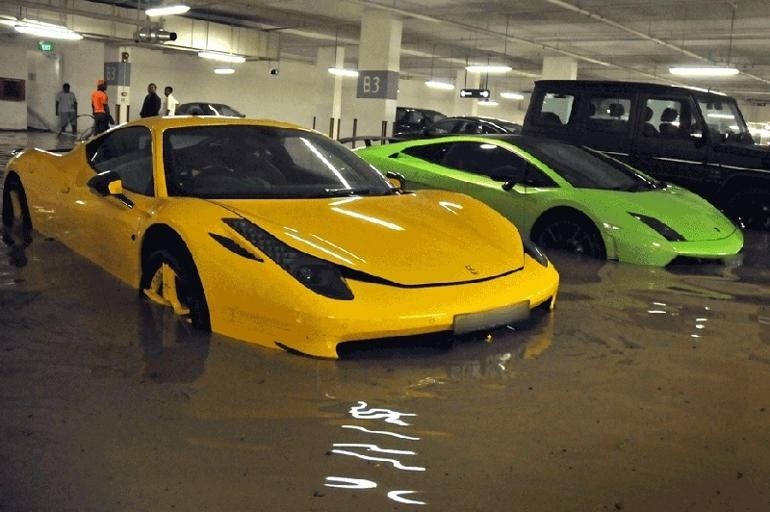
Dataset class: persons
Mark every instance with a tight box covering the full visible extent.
[163,87,179,116]
[140,83,161,118]
[91,79,111,135]
[55,83,78,135]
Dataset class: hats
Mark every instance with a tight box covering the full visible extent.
[97,79,105,86]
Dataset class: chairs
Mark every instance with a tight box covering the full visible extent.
[587,103,680,137]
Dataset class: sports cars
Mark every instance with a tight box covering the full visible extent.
[351,137,745,267]
[1,116,560,360]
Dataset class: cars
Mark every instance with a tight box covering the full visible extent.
[175,102,246,118]
[388,107,523,143]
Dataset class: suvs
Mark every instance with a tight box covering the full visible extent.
[520,80,770,233]
